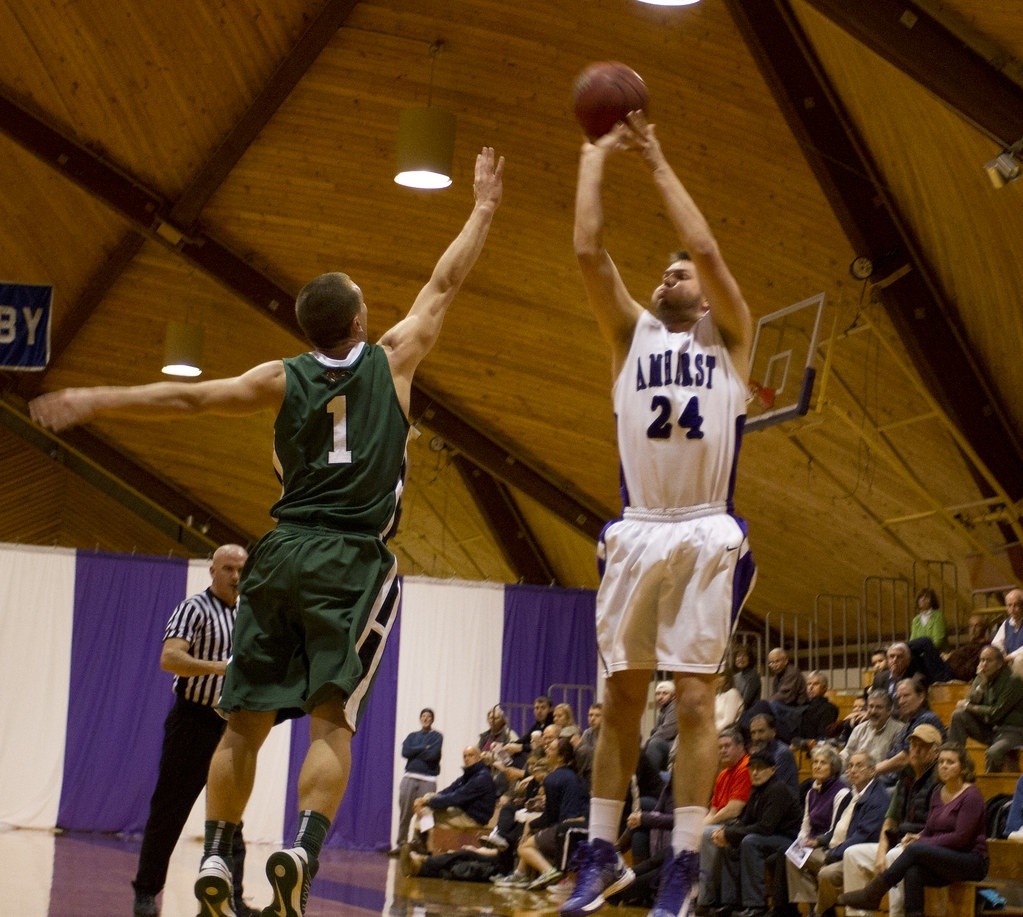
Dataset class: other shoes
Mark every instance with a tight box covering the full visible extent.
[733,907,770,917]
[837,889,880,910]
[704,905,737,917]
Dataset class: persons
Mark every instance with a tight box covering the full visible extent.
[29,148,504,917]
[390,696,662,891]
[133,544,262,917]
[562,108,750,917]
[618,589,1023,917]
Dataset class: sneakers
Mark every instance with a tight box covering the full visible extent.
[530,867,561,890]
[192,851,242,915]
[645,842,702,916]
[257,847,311,913]
[493,871,529,889]
[562,839,638,917]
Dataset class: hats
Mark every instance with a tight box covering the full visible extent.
[905,723,942,746]
[654,681,676,693]
[745,750,775,767]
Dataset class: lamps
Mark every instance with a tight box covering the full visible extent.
[159,300,203,377]
[850,254,873,280]
[409,420,421,439]
[392,38,458,189]
[985,137,1023,189]
[429,437,446,452]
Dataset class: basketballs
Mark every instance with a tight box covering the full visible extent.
[572,60,652,142]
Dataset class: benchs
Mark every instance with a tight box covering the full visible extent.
[795,604,1023,917]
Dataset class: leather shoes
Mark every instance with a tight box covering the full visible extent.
[133,893,156,916]
